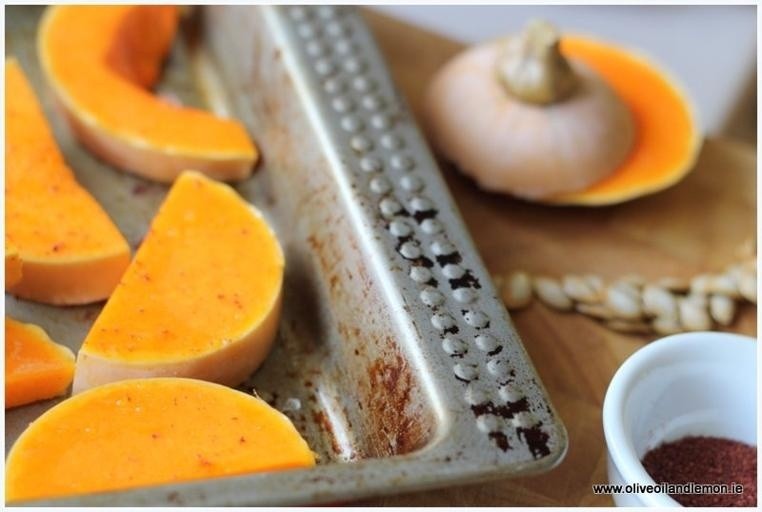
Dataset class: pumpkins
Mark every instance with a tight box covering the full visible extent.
[419,22,703,207]
[5,5,317,500]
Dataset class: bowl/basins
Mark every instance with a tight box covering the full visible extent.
[599,328,758,507]
